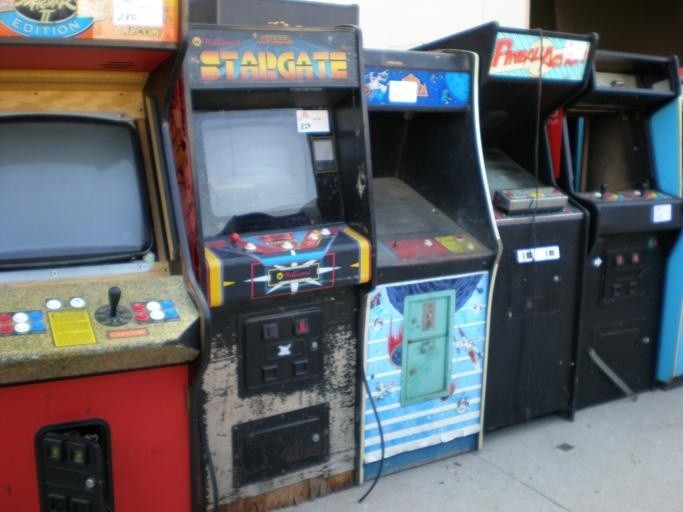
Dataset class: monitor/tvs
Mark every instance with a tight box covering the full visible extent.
[0,112,160,285]
[369,179,455,241]
[581,114,648,192]
[197,113,317,220]
[482,150,534,193]
